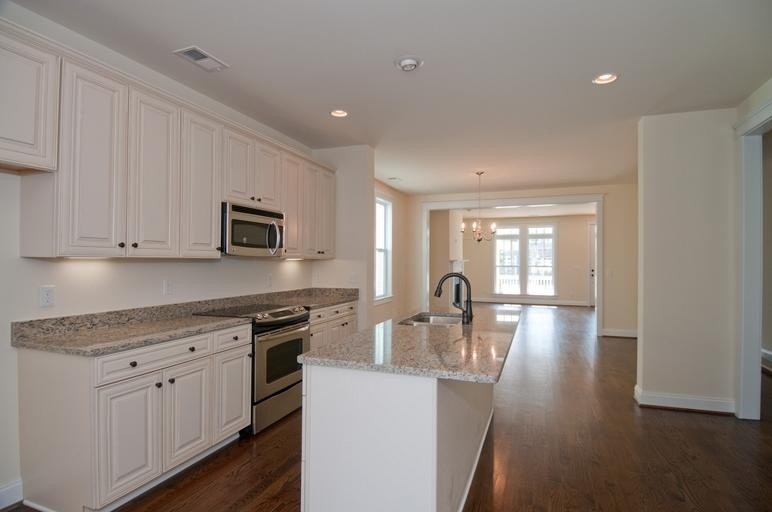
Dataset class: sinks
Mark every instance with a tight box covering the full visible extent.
[398,311,473,326]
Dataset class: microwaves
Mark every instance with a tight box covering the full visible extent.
[220,201,287,259]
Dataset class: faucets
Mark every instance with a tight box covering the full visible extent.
[433,325,473,372]
[434,272,473,322]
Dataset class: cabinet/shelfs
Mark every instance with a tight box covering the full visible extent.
[222,126,283,212]
[280,147,299,258]
[299,157,337,261]
[21,54,179,261]
[16,332,209,510]
[180,108,221,262]
[211,322,253,452]
[309,299,360,348]
[2,22,63,175]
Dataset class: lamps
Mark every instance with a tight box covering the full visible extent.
[458,172,497,247]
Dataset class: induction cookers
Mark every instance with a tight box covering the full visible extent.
[193,302,311,334]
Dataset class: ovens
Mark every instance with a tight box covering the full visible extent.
[252,321,310,434]
